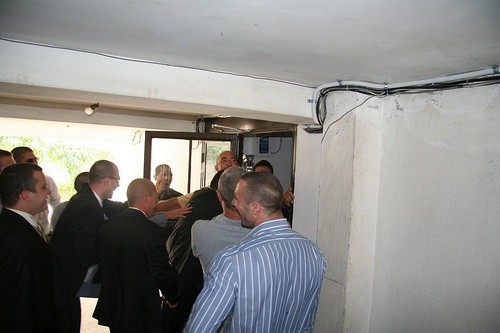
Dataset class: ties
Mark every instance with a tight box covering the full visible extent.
[37,221,48,244]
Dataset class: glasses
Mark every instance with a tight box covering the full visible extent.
[102,175,120,182]
[26,156,38,163]
[160,173,173,177]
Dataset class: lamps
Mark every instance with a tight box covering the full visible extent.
[84,103,100,116]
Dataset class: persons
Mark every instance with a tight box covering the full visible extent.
[92,178,193,333]
[192,167,252,292]
[283,187,294,207]
[182,172,326,333]
[11,146,60,240]
[0,162,81,300]
[45,171,98,241]
[215,150,240,171]
[160,170,224,333]
[49,160,130,285]
[252,160,275,177]
[152,164,184,200]
[0,149,16,171]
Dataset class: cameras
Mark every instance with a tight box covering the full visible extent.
[243,154,254,172]
